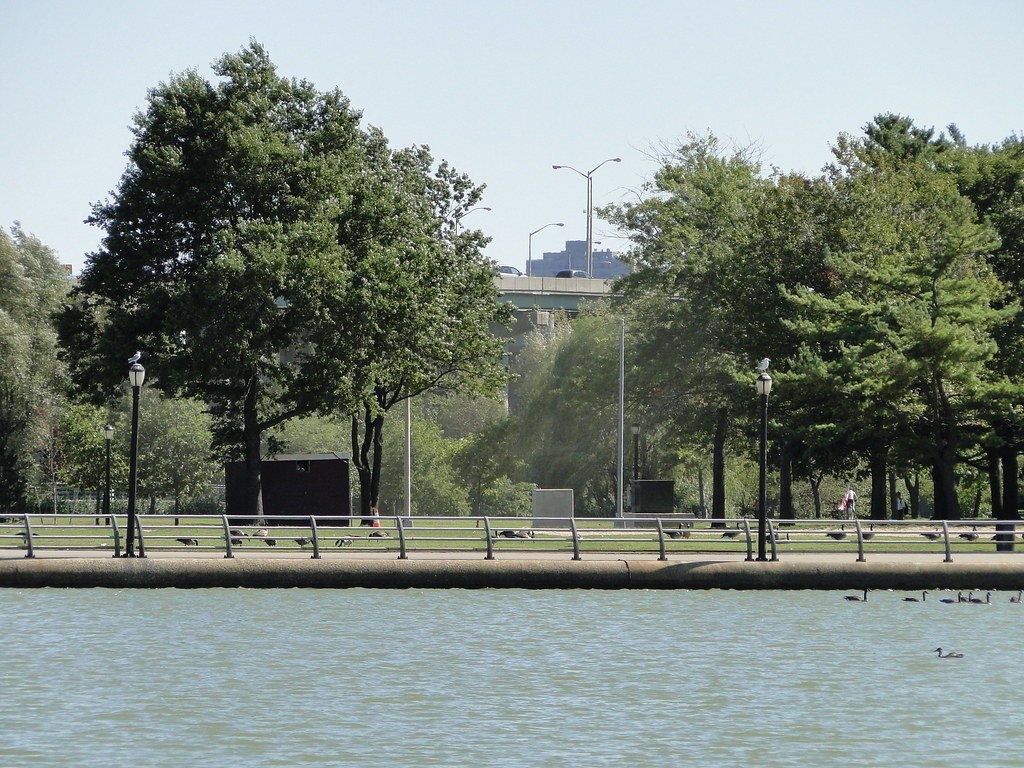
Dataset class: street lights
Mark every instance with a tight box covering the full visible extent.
[102,423,116,525]
[552,156,623,278]
[122,351,147,560]
[453,205,492,236]
[527,221,564,276]
[753,357,774,563]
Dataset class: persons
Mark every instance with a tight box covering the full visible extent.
[844,487,858,520]
[896,491,906,520]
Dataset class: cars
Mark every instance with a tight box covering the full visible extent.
[556,268,592,277]
[493,265,525,278]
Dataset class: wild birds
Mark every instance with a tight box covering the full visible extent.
[843,587,872,602]
[939,591,963,604]
[663,522,691,539]
[826,523,846,540]
[252,522,269,537]
[766,526,791,540]
[492,530,535,547]
[991,535,996,541]
[1010,590,1024,603]
[958,526,979,542]
[224,529,251,547]
[265,539,276,547]
[174,539,198,547]
[292,539,313,547]
[334,534,361,547]
[919,528,941,541]
[960,591,973,603]
[901,591,930,602]
[368,532,387,542]
[721,523,742,540]
[933,648,966,658]
[863,524,875,540]
[971,592,992,604]
